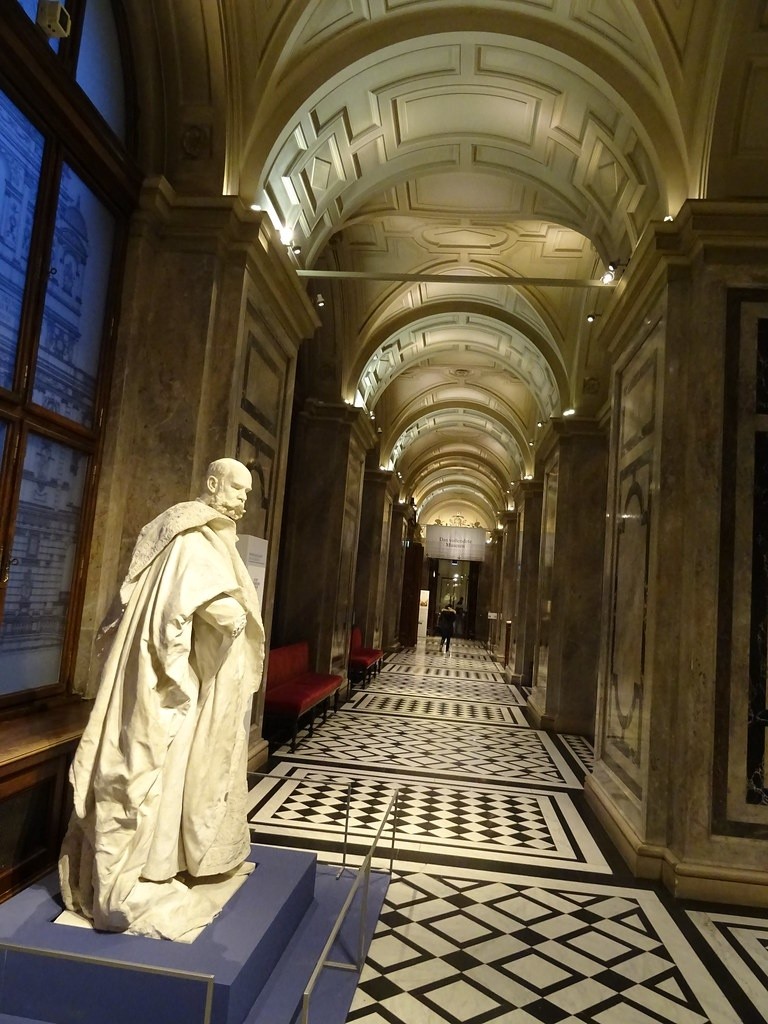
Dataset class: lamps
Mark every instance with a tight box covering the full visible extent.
[608,257,628,272]
[538,419,548,427]
[311,294,325,307]
[285,238,301,254]
[587,310,602,321]
[529,439,539,445]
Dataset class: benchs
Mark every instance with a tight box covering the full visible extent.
[262,642,343,754]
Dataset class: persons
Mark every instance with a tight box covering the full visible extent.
[57,457,265,933]
[438,604,456,658]
[454,597,465,635]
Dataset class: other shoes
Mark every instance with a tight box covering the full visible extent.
[438,645,443,652]
[444,651,450,658]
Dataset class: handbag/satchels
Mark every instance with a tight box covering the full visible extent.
[436,612,442,628]
